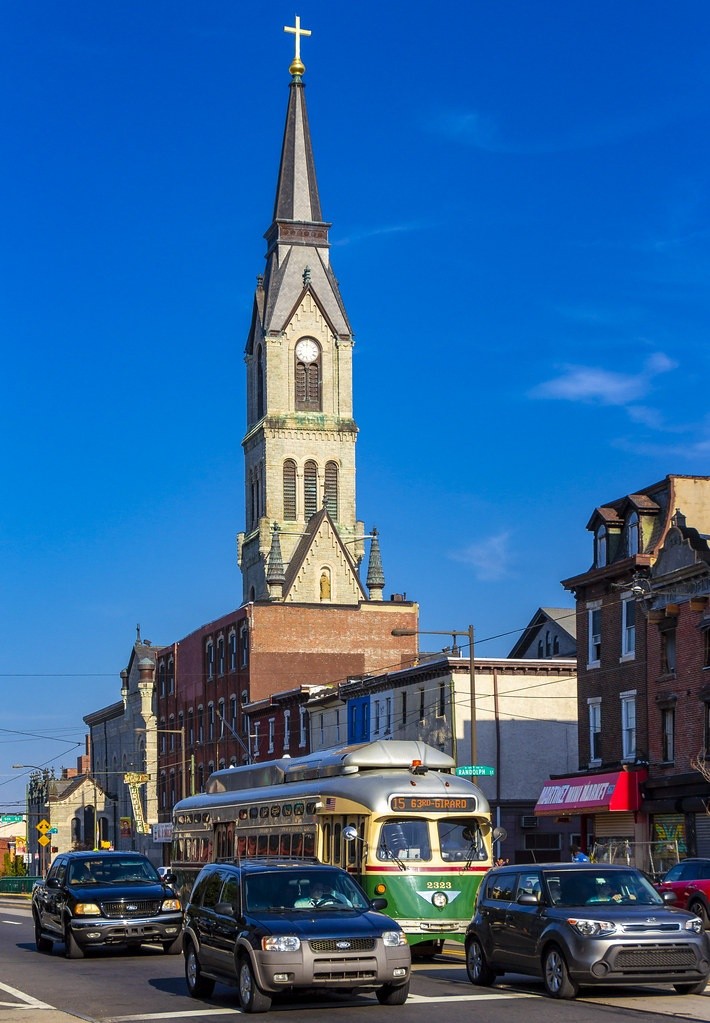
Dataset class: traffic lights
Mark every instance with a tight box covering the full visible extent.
[52,847,58,852]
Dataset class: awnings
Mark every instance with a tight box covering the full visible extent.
[533,771,642,816]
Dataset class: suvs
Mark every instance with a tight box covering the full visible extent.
[31,849,183,959]
[463,861,710,1000]
[180,854,415,1013]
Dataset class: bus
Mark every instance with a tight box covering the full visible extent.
[169,738,508,963]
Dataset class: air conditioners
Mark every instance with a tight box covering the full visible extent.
[520,815,537,827]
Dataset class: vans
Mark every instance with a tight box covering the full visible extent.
[636,857,710,930]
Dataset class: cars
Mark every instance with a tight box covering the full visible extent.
[158,866,172,890]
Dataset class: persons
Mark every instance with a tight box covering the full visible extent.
[294,877,354,908]
[570,845,589,862]
[495,857,510,866]
[71,866,96,883]
[438,826,464,851]
[585,883,636,903]
[120,821,130,834]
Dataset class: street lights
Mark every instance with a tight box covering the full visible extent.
[135,726,185,801]
[390,624,481,784]
[12,764,51,873]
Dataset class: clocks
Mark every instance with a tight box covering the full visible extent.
[295,340,319,363]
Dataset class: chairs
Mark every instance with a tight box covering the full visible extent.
[248,879,273,910]
